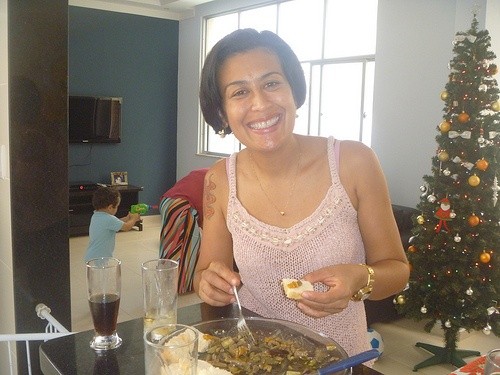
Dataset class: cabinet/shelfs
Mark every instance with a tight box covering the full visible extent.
[69,183,145,237]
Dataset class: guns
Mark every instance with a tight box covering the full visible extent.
[130,204,162,213]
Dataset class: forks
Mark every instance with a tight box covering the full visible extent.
[231,285,258,347]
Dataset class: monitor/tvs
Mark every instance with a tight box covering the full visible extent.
[69,94,122,143]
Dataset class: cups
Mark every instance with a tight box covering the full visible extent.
[141,259,179,340]
[86,256,123,350]
[144,324,198,375]
[484,348,500,375]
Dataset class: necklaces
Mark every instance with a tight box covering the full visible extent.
[245,135,302,216]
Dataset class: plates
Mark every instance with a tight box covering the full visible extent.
[152,316,351,375]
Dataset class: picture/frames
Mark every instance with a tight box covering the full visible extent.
[111,171,128,185]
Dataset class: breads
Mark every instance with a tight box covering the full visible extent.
[282,278,314,300]
[159,325,234,375]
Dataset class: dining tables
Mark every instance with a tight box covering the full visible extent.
[39,296,384,375]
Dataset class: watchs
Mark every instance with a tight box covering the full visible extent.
[351,262,375,302]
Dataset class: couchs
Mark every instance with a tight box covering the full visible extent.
[364,203,424,325]
[159,166,208,297]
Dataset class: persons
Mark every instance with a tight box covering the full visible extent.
[192,27,410,368]
[84,187,140,266]
